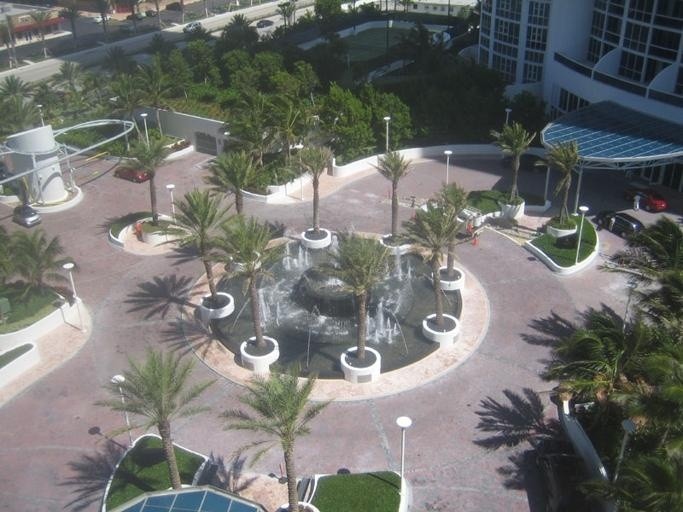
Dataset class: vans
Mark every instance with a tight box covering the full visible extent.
[182,21,202,32]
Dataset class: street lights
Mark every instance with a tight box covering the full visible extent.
[108,373,136,448]
[392,414,414,495]
[136,110,152,152]
[610,416,639,483]
[574,204,590,265]
[383,12,395,65]
[36,102,47,126]
[441,147,454,185]
[59,258,90,337]
[382,115,392,155]
[503,107,514,124]
[163,179,182,245]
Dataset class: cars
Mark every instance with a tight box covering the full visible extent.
[89,8,160,37]
[534,449,593,512]
[256,20,273,29]
[12,203,43,229]
[165,1,181,11]
[32,86,66,102]
[620,183,668,213]
[114,163,154,184]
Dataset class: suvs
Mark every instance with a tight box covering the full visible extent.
[592,207,645,240]
